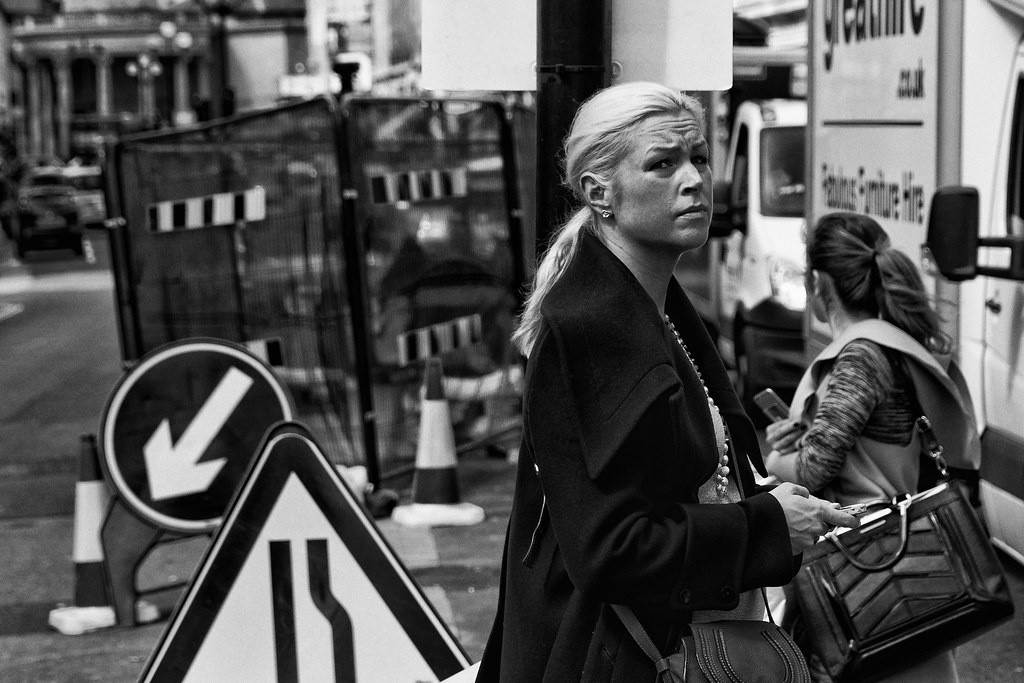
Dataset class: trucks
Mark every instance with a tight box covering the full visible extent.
[801,0,1024,566]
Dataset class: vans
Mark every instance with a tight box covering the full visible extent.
[708,97,807,372]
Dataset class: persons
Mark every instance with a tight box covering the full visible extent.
[766,211,981,683]
[475,83,858,683]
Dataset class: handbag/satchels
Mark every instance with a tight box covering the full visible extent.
[792,345,1013,683]
[653,619,812,683]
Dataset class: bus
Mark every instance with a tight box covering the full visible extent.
[2,165,105,240]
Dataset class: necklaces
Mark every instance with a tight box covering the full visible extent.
[665,313,730,498]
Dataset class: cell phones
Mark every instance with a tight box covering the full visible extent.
[835,504,867,515]
[753,388,789,423]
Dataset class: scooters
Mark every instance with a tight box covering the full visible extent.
[14,185,87,265]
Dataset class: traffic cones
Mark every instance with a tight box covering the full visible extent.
[390,358,488,530]
[47,433,163,638]
